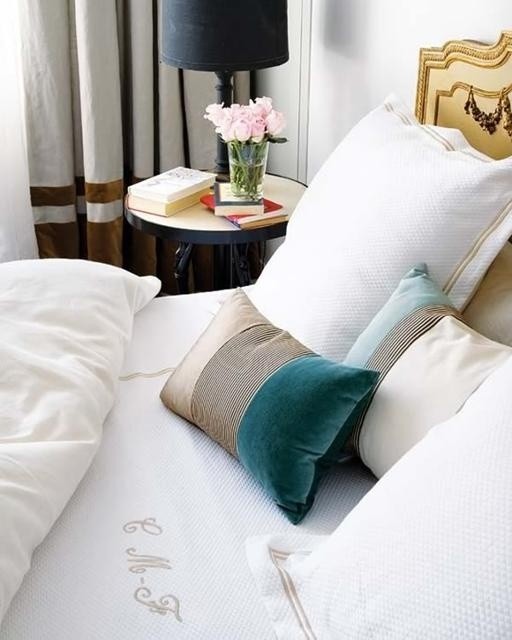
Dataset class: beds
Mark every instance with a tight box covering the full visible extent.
[0,28,511,640]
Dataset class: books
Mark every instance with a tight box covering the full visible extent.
[127,166,289,229]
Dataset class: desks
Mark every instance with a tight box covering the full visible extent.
[122,165,311,296]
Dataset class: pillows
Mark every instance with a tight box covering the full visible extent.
[159,288,379,525]
[244,354,510,639]
[242,91,511,365]
[342,262,510,480]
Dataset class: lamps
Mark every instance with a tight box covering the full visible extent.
[158,1,292,182]
